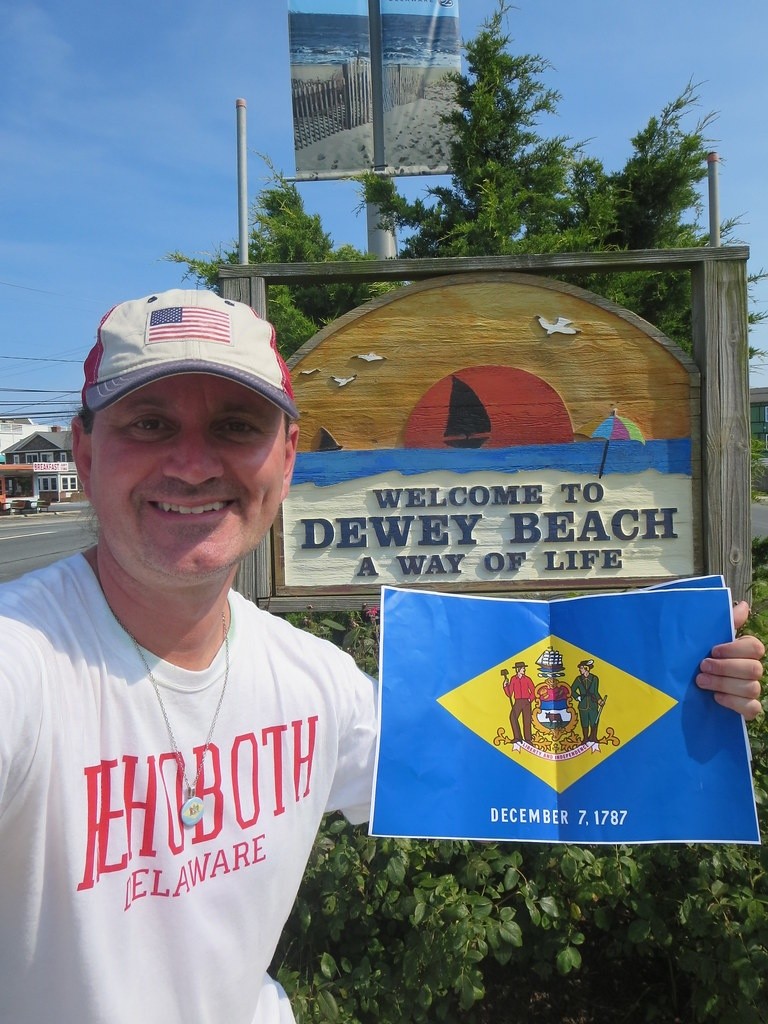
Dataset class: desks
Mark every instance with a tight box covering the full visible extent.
[3,501,18,515]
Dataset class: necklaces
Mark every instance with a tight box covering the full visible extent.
[116,613,229,827]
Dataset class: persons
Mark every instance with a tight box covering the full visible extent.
[0,287,764,1024]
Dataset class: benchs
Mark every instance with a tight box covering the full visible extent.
[36,502,50,514]
[8,507,24,515]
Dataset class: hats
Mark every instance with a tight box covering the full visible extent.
[82,288,299,422]
